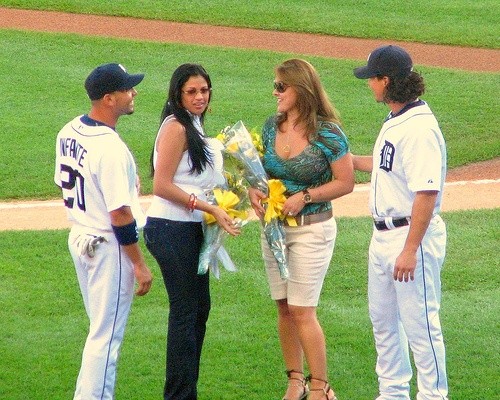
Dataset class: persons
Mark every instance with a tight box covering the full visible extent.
[143,64,241,400]
[248,58,355,400]
[54,64,153,400]
[351,45,449,400]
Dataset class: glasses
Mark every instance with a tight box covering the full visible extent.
[180,87,213,97]
[273,81,290,94]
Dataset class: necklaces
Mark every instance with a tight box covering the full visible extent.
[282,145,293,152]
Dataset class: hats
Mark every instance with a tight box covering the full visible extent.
[85,63,144,100]
[353,45,413,80]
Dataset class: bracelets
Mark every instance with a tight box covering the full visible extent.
[185,194,197,213]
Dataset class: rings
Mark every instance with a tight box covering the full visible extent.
[287,212,292,216]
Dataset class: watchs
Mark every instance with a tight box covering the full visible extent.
[302,190,311,204]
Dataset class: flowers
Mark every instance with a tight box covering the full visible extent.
[197,122,298,280]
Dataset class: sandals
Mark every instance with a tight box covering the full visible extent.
[309,373,337,400]
[283,369,310,400]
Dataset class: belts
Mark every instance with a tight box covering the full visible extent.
[374,216,412,230]
